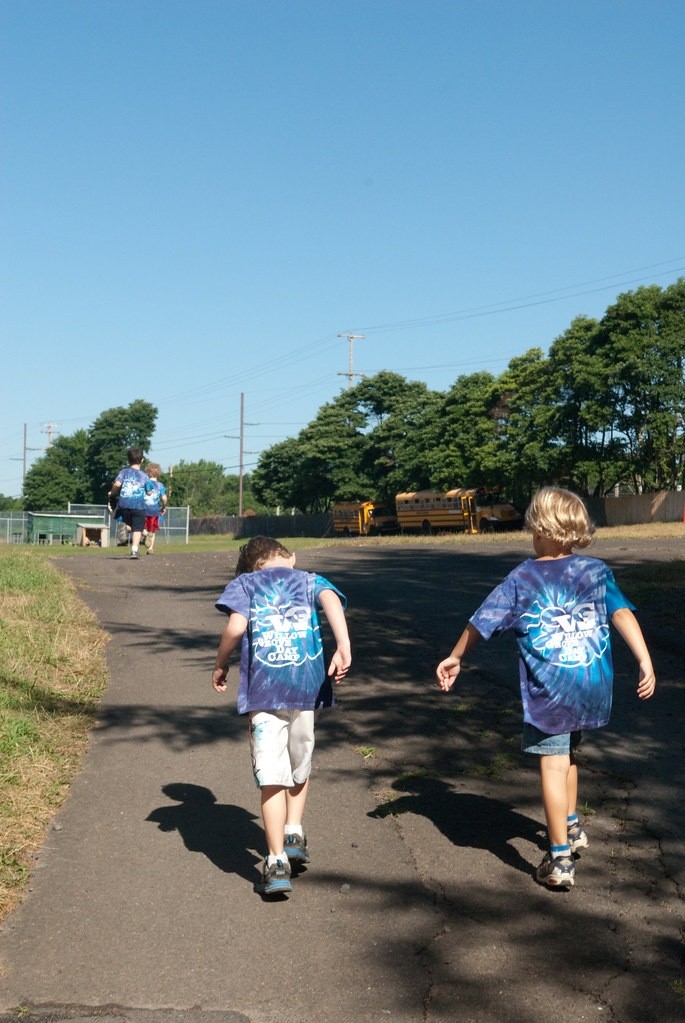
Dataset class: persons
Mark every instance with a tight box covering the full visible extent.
[212,536,351,894]
[436,488,657,886]
[108,448,154,559]
[141,464,167,555]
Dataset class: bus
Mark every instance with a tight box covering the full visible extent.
[395,483,522,534]
[332,499,396,537]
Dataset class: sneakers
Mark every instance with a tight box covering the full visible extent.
[566,823,590,854]
[283,832,310,864]
[536,848,575,886]
[256,857,292,894]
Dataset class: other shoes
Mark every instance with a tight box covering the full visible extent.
[147,549,153,556]
[130,551,141,559]
